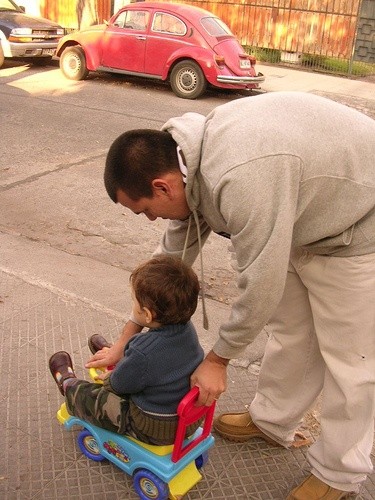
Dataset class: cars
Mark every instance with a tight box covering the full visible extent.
[54,1,266,101]
[0,1,67,68]
[58,367,215,500]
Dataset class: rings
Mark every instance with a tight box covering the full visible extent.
[214,397,219,401]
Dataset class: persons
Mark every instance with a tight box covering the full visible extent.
[49,251,204,446]
[104,92,375,500]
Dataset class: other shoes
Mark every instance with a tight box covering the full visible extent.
[88,333,111,353]
[48,350,77,396]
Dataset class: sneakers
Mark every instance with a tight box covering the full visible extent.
[213,409,291,447]
[284,473,359,500]
